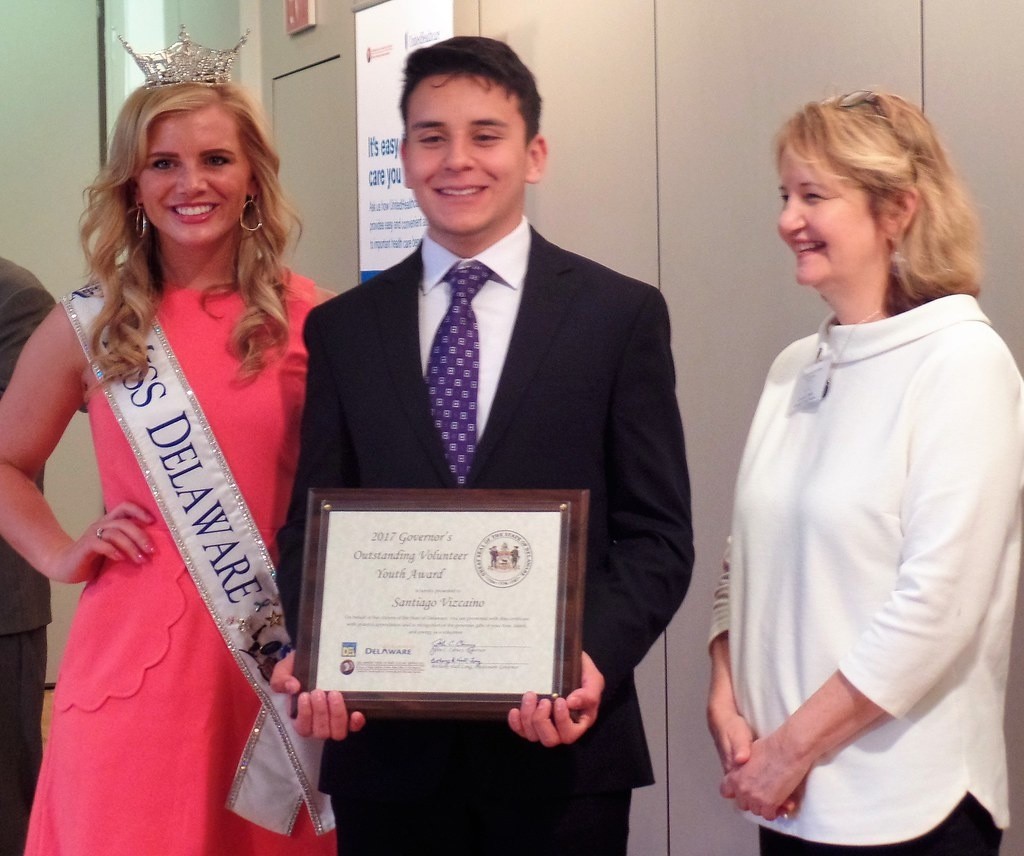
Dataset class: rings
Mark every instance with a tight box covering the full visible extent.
[97,527,104,537]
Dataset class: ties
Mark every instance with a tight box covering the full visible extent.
[423,262,492,487]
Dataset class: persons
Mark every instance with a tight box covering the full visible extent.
[0,255,61,854]
[270,36,694,856]
[0,77,343,856]
[707,88,1024,856]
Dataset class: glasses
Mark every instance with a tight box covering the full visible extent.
[819,90,918,185]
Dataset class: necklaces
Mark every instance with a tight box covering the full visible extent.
[821,310,880,399]
